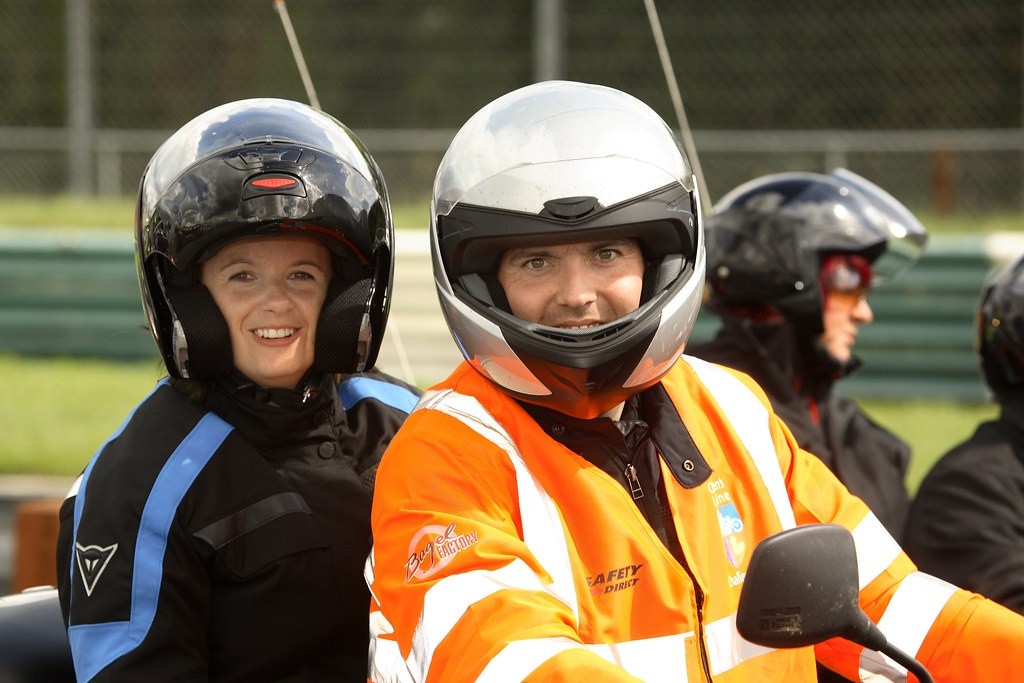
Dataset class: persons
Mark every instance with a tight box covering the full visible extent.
[57,96,423,682]
[364,81,1024,682]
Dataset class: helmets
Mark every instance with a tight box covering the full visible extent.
[134,99,395,387]
[979,256,1024,406]
[429,81,706,419]
[702,171,888,339]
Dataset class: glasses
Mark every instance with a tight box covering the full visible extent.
[818,252,875,295]
